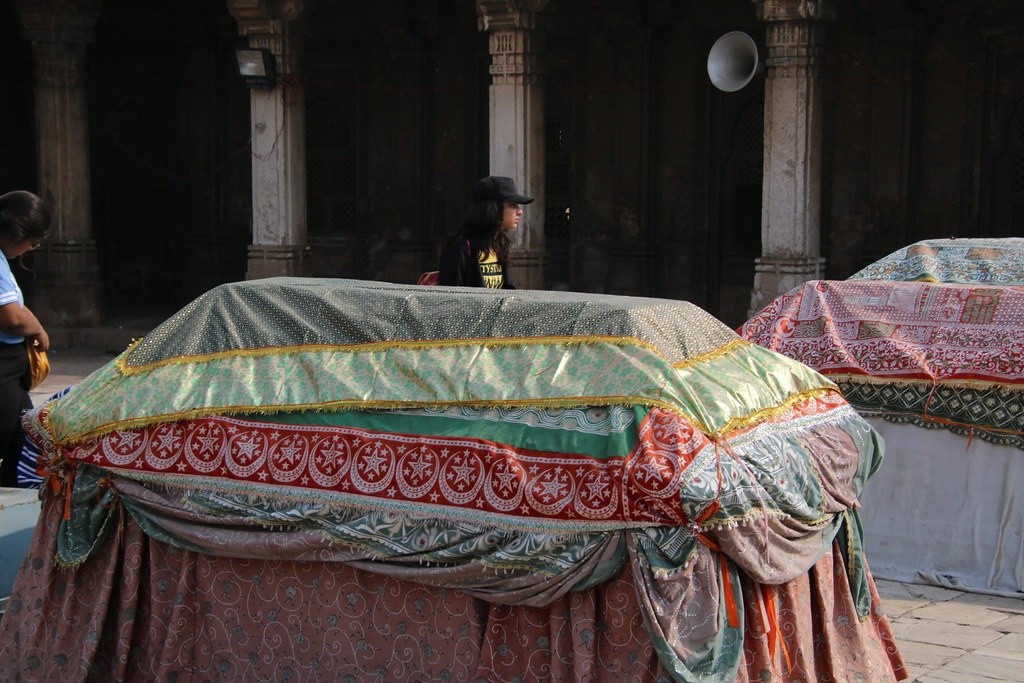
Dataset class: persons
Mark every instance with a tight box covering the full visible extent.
[439,176,534,288]
[0,191,52,488]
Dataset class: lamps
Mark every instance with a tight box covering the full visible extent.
[232,45,278,91]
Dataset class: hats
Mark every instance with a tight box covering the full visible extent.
[477,176,534,205]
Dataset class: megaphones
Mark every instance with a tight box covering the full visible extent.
[707,31,768,91]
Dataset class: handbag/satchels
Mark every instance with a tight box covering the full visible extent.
[23,338,50,393]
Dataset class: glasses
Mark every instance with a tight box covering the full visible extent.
[29,241,41,250]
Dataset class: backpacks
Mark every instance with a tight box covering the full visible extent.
[416,233,470,285]
